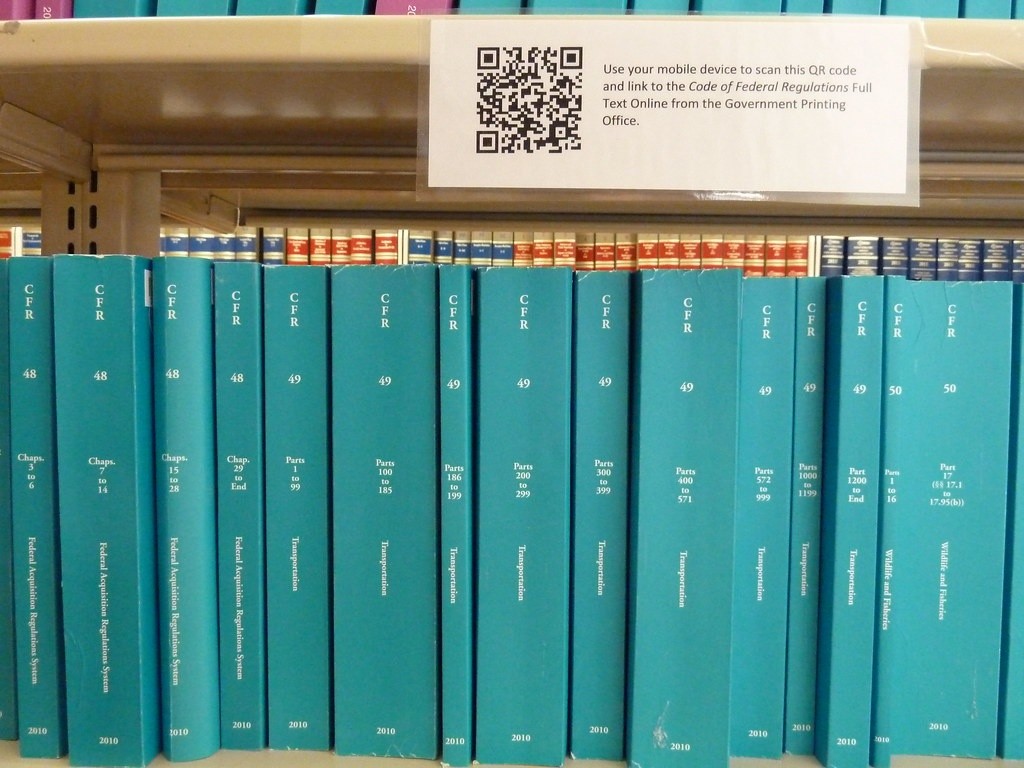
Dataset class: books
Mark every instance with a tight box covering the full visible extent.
[0,228,1024,768]
[0,0,1024,20]
